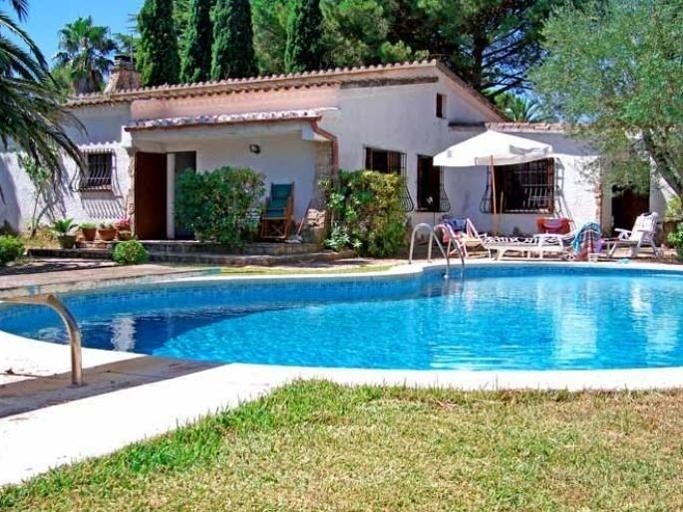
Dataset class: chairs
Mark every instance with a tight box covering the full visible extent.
[259,181,295,240]
[438,211,662,260]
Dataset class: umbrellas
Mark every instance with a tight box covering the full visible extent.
[433,129,553,236]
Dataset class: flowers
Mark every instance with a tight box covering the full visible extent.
[113,219,132,229]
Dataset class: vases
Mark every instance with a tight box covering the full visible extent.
[117,230,130,241]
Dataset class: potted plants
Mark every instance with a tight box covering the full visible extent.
[45,216,119,250]
